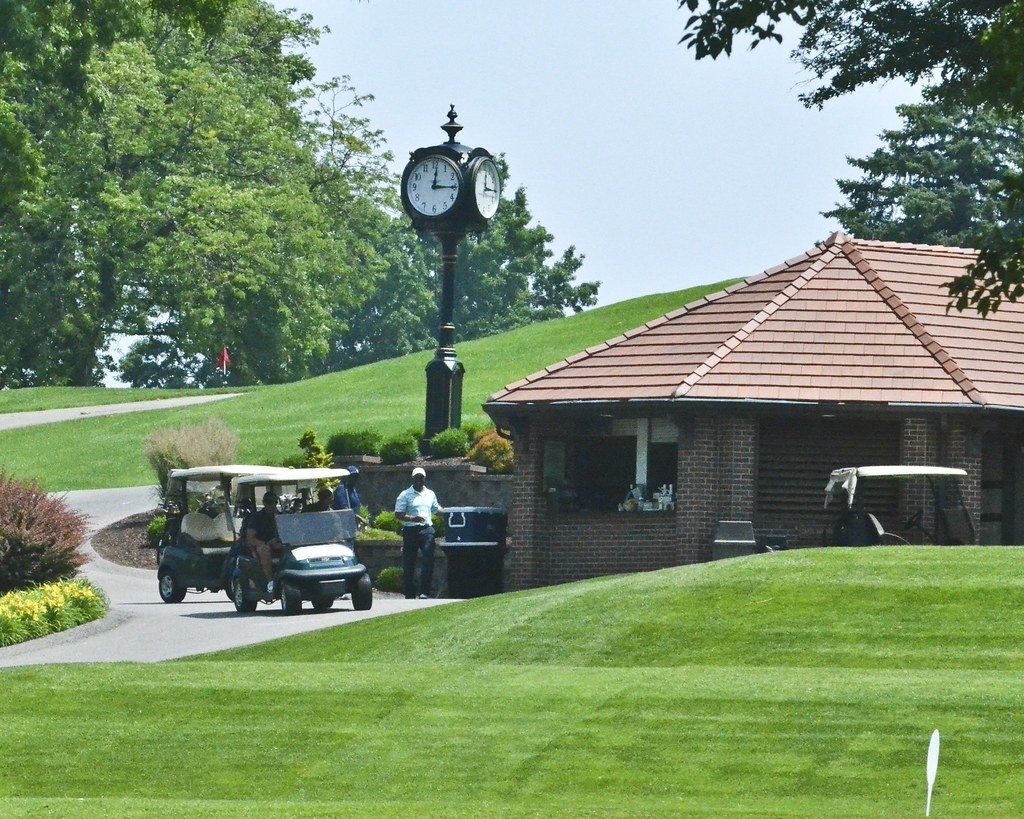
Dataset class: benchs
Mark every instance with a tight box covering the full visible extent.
[180,510,243,556]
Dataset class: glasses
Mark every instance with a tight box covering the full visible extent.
[267,500,278,504]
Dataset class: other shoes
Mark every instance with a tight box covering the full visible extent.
[266,580,274,593]
[420,593,426,598]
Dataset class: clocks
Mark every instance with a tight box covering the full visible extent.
[401,151,463,222]
[465,156,502,221]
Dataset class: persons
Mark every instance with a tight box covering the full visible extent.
[395,468,444,599]
[245,465,367,600]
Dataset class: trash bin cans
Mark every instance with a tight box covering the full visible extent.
[712,518,757,562]
[440,504,507,599]
[762,535,788,552]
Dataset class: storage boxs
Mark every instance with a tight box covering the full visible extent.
[443,506,507,543]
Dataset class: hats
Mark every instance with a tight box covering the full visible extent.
[347,466,359,474]
[411,468,427,477]
